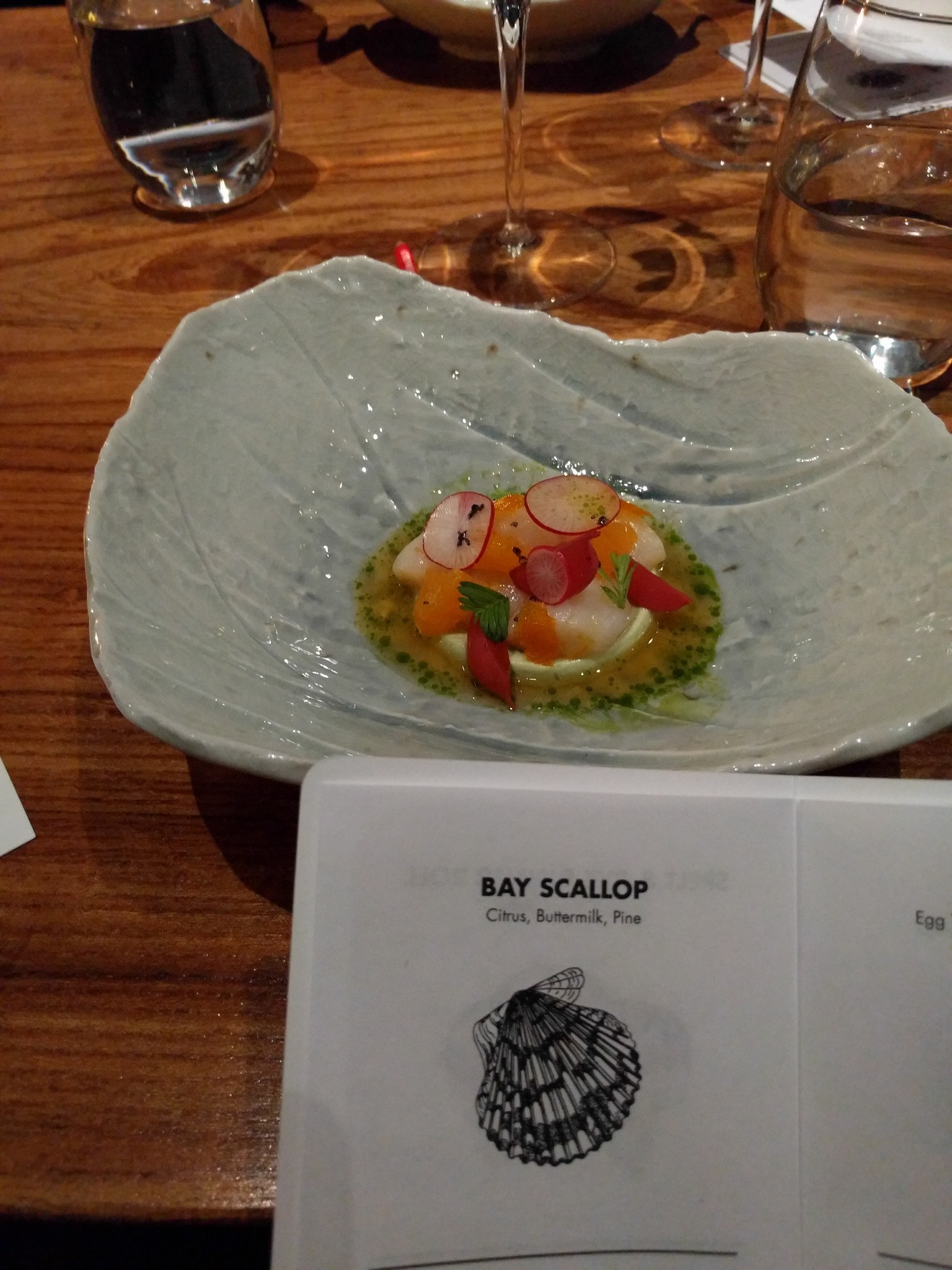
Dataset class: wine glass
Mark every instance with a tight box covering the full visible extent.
[658,0,789,172]
[418,0,617,311]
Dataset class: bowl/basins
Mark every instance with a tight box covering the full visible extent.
[373,0,661,64]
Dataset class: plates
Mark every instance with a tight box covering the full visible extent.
[84,254,952,784]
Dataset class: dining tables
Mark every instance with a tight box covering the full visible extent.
[0,0,952,1270]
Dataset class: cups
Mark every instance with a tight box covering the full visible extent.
[66,0,284,209]
[751,0,952,400]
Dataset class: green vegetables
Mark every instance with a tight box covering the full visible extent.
[596,552,634,608]
[455,581,510,643]
